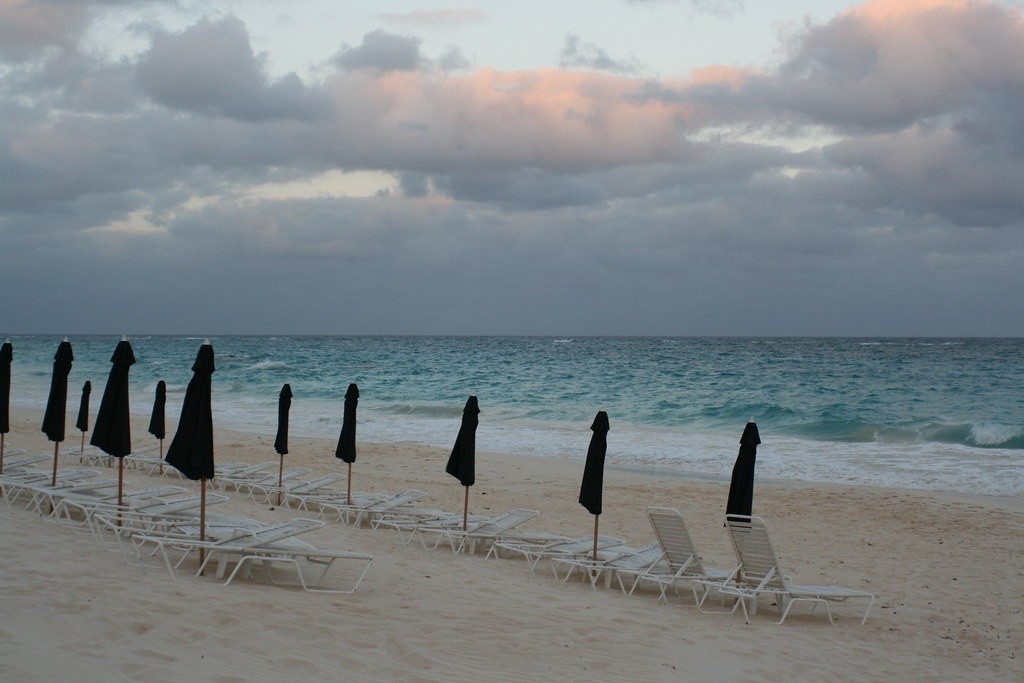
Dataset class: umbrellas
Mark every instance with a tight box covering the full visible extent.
[42,336,73,516]
[0,338,13,499]
[444,390,480,540]
[76,377,92,462]
[90,335,137,532]
[723,416,761,609]
[148,377,167,476]
[164,338,215,575]
[335,378,360,505]
[579,406,610,586]
[273,380,294,507]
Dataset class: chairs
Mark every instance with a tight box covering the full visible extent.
[0,427,887,627]
